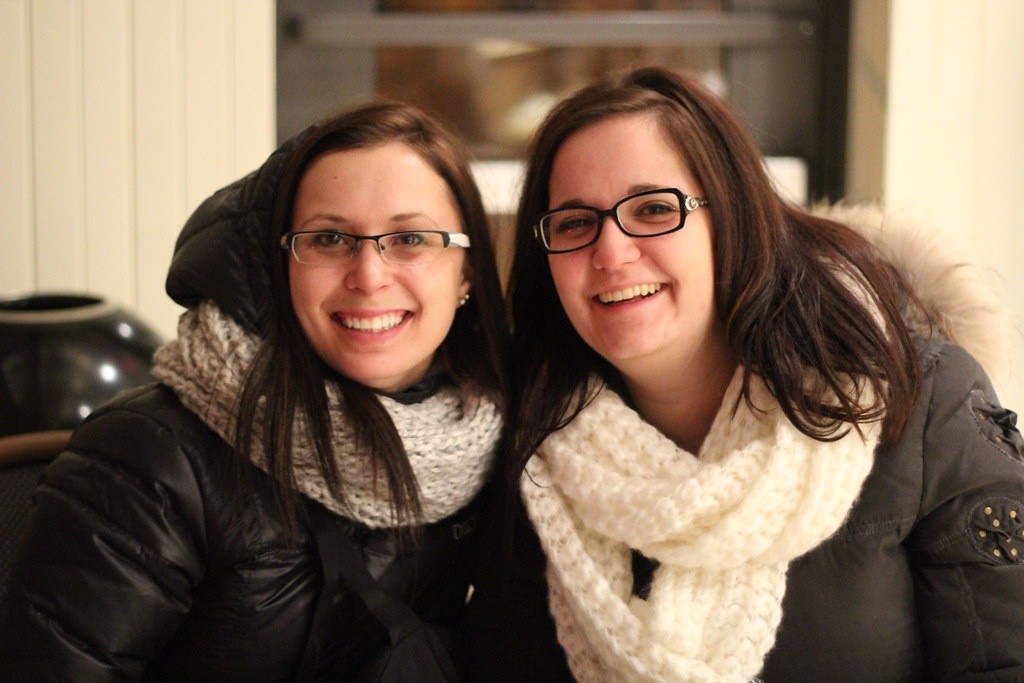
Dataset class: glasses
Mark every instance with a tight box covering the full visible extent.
[532,189,710,254]
[280,229,471,265]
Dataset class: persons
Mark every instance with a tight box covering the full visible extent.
[0,101,513,683]
[493,66,1024,682]
